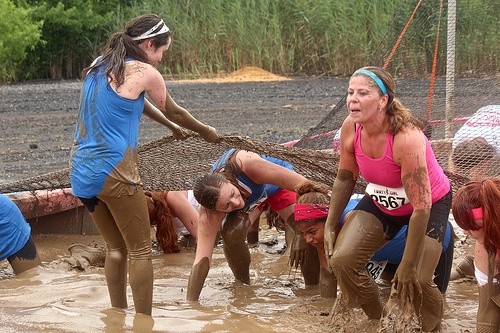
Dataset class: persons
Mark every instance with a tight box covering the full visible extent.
[0,195,107,275]
[145,190,259,253]
[68,13,222,316]
[294,180,474,317]
[452,176,500,333]
[448,105,500,181]
[186,147,320,301]
[323,66,453,333]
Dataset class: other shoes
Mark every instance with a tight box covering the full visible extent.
[68,244,105,266]
[459,255,475,276]
